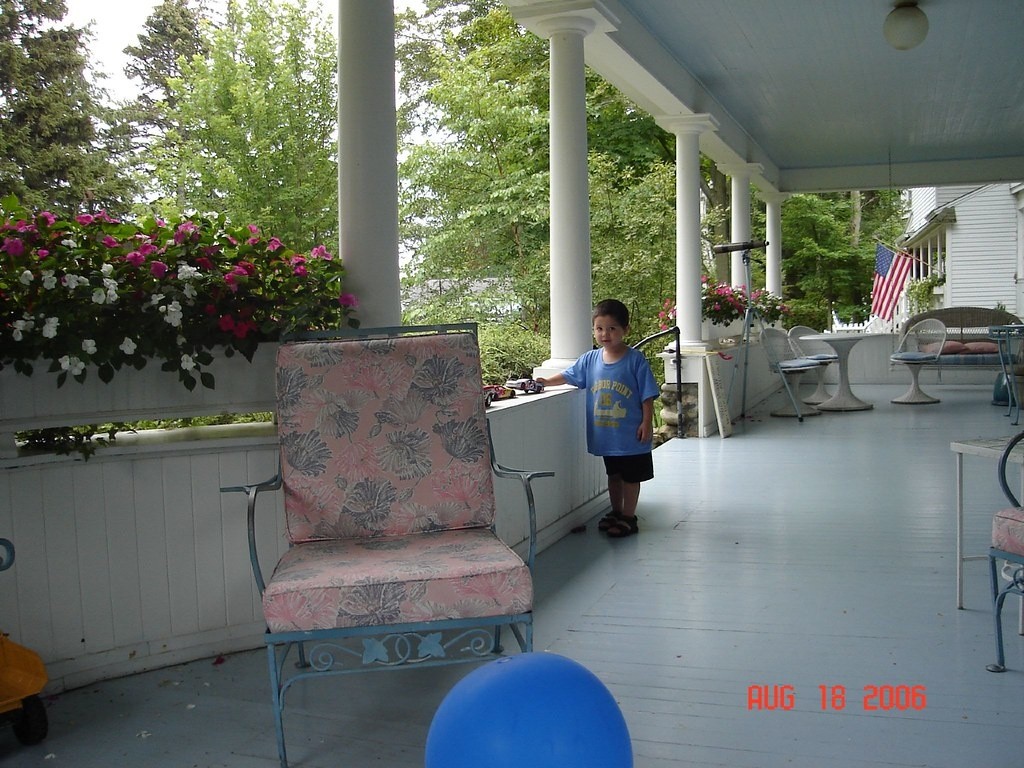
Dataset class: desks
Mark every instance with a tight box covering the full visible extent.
[988,325,1024,425]
[950,436,1024,634]
[799,333,884,412]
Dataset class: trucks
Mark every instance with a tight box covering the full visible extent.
[483,386,495,409]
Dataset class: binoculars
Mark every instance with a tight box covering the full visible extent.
[713,240,769,254]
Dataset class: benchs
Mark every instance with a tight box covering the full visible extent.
[899,306,1024,382]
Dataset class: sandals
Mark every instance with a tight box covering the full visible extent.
[599,510,624,531]
[607,515,639,537]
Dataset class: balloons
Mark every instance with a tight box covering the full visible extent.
[424,652,634,768]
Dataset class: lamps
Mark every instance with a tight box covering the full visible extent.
[883,0,929,50]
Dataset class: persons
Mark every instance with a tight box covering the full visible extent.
[537,298,660,538]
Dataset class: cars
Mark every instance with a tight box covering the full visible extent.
[506,376,544,394]
[495,385,516,400]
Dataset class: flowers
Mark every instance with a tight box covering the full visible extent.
[658,274,794,331]
[0,211,361,394]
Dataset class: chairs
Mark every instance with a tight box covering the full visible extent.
[759,327,823,416]
[889,319,947,404]
[789,326,839,405]
[987,429,1024,673]
[221,323,556,768]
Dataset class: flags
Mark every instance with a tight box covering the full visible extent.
[870,243,913,321]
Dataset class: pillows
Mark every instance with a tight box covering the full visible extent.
[960,342,1001,355]
[924,341,968,354]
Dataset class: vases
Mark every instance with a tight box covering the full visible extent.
[701,315,744,341]
